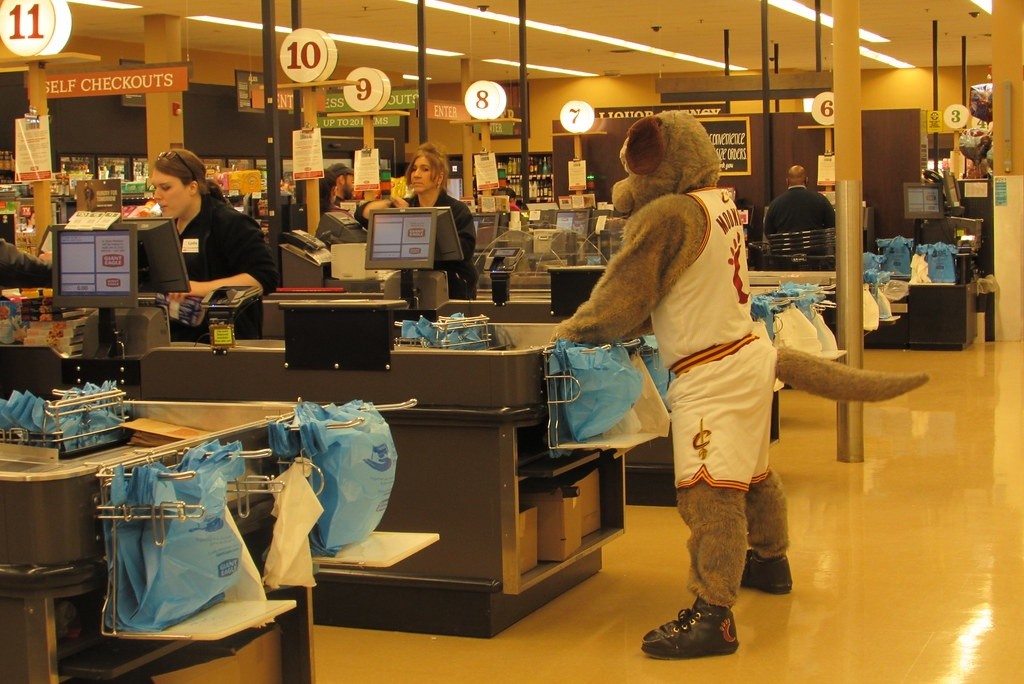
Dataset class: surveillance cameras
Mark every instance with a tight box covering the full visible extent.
[968,12,980,18]
[652,26,661,31]
[477,5,489,12]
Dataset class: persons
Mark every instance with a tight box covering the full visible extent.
[320,169,353,218]
[0,238,54,289]
[498,186,520,210]
[355,143,478,300]
[766,165,835,268]
[327,162,353,206]
[150,149,283,341]
[83,182,91,211]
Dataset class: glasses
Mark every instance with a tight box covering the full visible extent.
[158,151,196,180]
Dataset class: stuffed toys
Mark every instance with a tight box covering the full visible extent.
[551,112,929,662]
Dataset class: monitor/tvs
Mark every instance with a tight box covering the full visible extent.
[956,178,994,208]
[52,217,191,309]
[903,183,944,219]
[364,206,610,270]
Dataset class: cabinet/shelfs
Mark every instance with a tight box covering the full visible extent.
[473,151,555,208]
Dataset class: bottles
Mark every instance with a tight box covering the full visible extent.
[258,194,268,217]
[529,157,552,174]
[587,171,595,194]
[529,174,554,203]
[261,165,268,193]
[229,162,239,197]
[21,287,53,298]
[518,486,581,497]
[507,156,521,175]
[217,166,226,198]
[208,318,237,349]
[56,175,76,197]
[122,197,162,218]
[133,160,148,182]
[61,157,90,174]
[506,175,523,198]
[497,162,507,178]
[0,151,15,184]
[98,158,125,180]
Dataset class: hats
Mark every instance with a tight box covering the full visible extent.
[328,163,353,176]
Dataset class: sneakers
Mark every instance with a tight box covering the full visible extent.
[741,550,792,594]
[641,598,738,659]
